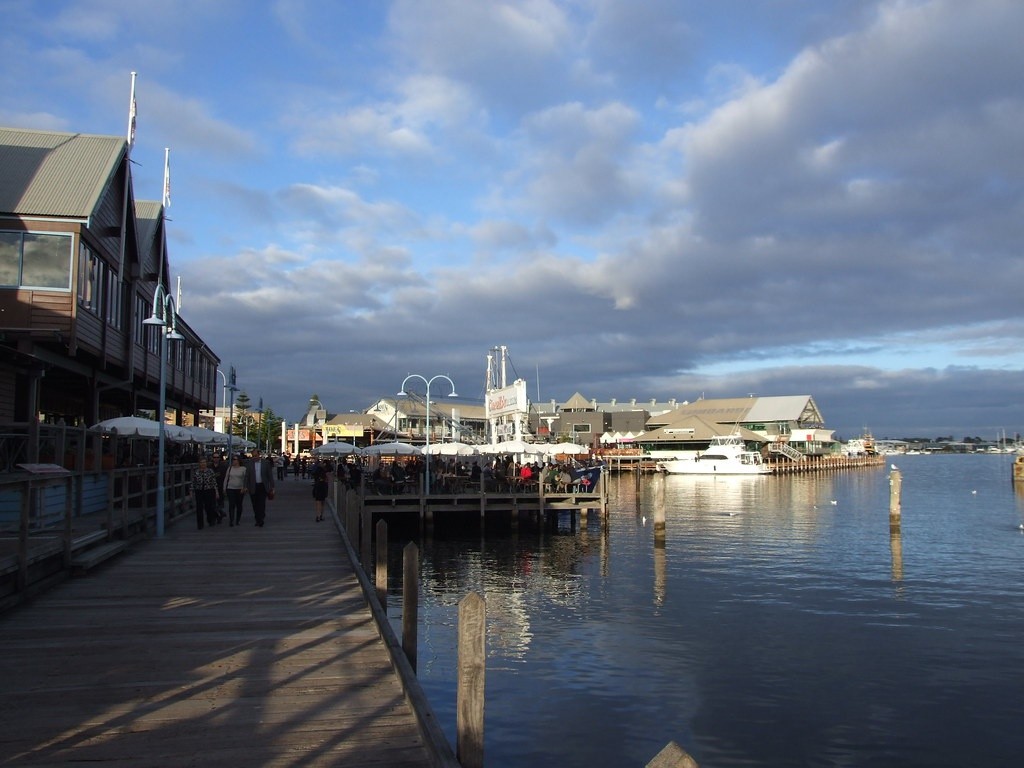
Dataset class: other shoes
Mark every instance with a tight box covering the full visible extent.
[255,521,260,527]
[229,520,234,528]
[320,515,324,521]
[236,520,240,526]
[197,525,204,530]
[208,522,217,527]
[259,520,265,528]
[302,477,306,479]
[315,516,320,522]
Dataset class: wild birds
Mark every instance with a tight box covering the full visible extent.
[728,513,736,515]
[971,490,976,494]
[813,506,817,509]
[829,501,837,505]
[643,516,647,521]
[1018,524,1024,530]
[890,464,900,471]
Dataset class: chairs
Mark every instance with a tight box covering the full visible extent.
[361,471,587,496]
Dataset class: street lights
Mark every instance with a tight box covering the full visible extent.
[238,415,255,453]
[142,284,186,537]
[350,409,364,425]
[309,398,323,410]
[395,374,459,496]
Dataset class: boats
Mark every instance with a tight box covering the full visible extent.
[881,448,930,455]
[656,409,775,475]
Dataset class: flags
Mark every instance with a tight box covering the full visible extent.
[132,93,136,148]
[166,159,170,207]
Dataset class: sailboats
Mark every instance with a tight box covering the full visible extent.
[973,428,1024,455]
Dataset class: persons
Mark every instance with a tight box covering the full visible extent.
[118,442,197,505]
[276,453,290,481]
[336,456,571,492]
[222,454,248,527]
[208,453,227,507]
[266,454,273,469]
[308,458,333,522]
[243,449,275,527]
[192,457,219,530]
[302,456,308,479]
[294,455,301,478]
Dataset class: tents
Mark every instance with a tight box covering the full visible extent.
[601,431,645,448]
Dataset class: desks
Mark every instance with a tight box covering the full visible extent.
[444,475,470,494]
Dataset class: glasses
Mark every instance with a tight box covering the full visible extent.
[213,457,219,458]
[231,458,237,460]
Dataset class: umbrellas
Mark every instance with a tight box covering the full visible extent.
[91,416,255,466]
[310,440,361,456]
[364,440,589,462]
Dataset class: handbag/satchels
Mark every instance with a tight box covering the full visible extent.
[267,488,275,501]
